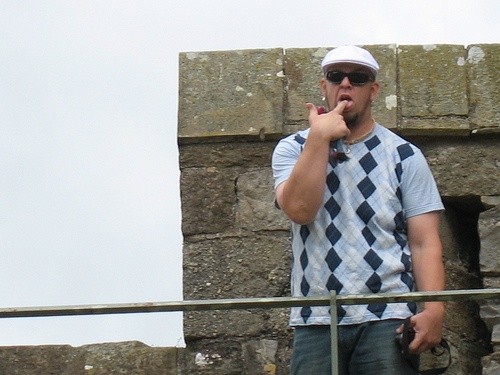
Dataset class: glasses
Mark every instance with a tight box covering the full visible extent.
[324,70,374,85]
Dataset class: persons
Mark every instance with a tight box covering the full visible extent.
[271,46,448,375]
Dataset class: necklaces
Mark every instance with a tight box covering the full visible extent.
[342,116,376,154]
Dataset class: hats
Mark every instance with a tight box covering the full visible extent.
[320,46,380,80]
